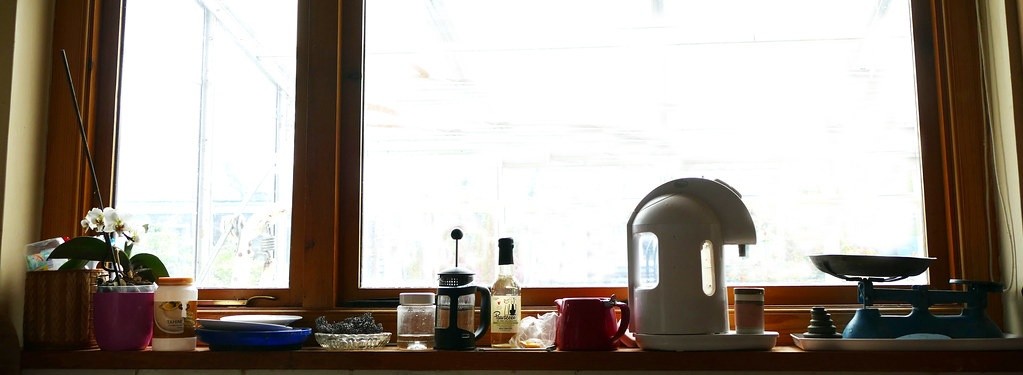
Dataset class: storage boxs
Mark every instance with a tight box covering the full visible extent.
[23,269,106,349]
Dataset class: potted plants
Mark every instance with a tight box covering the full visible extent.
[91,283,155,352]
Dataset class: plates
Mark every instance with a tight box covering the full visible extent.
[316,331,392,350]
[194,314,313,348]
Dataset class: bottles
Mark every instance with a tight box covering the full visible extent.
[733,287,765,334]
[490,238,521,348]
[397,292,436,349]
[152,277,198,351]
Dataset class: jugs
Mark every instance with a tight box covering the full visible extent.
[434,267,491,350]
[553,294,631,351]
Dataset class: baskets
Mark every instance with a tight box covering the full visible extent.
[22,266,104,349]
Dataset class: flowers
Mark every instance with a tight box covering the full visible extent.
[46,206,170,285]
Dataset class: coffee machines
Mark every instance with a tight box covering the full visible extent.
[627,175,780,350]
